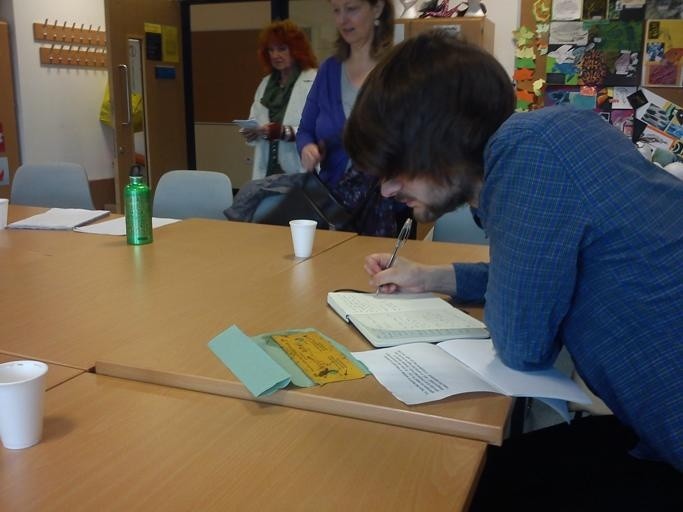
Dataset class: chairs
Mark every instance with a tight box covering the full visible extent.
[10,161,233,220]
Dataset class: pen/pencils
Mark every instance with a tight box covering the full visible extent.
[375,218,413,296]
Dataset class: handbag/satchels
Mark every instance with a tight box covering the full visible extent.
[257,174,353,230]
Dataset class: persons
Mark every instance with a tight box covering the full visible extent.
[294,0,397,239]
[342,28,682,511]
[235,18,318,183]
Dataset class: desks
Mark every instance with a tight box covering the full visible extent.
[0,201,519,510]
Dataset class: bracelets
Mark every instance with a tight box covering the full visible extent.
[283,126,290,141]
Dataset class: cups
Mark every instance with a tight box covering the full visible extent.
[0,360,48,450]
[288,219,318,259]
[0,199,9,231]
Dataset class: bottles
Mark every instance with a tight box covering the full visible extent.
[122,165,153,246]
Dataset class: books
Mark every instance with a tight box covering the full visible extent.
[326,290,492,348]
[7,207,109,231]
